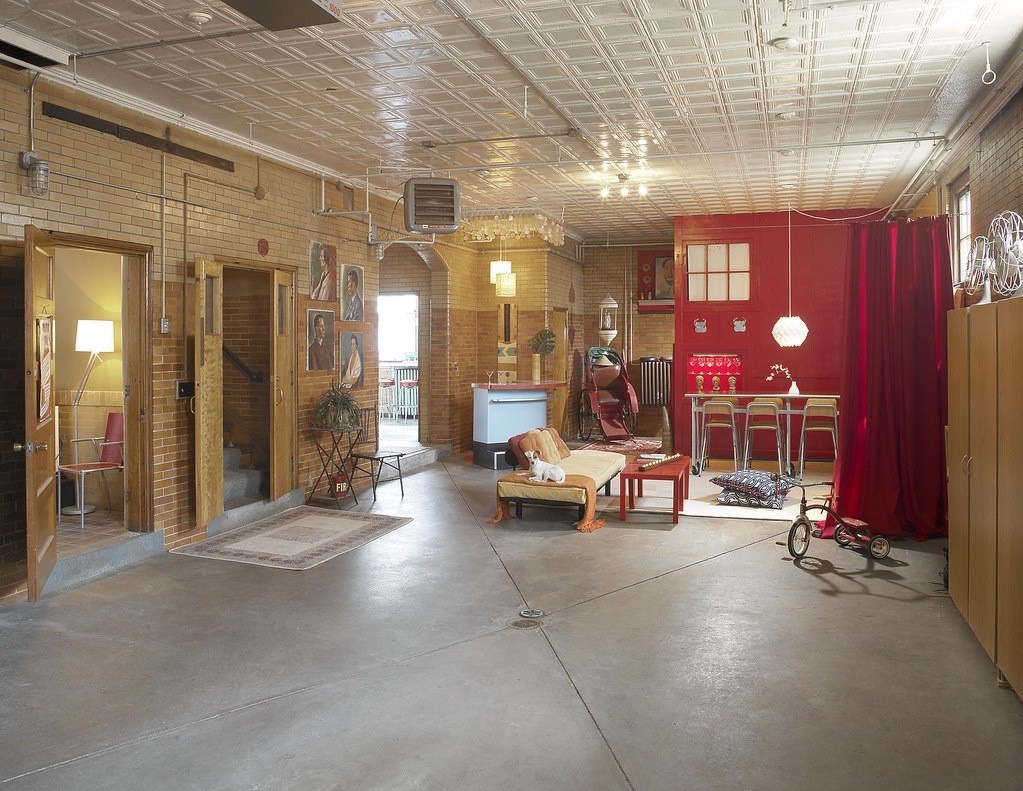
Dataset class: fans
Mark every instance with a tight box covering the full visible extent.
[964,236,997,308]
[985,210,1023,297]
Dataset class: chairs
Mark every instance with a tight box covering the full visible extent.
[347,404,406,501]
[58,413,124,529]
[742,397,786,475]
[698,397,742,478]
[796,398,838,482]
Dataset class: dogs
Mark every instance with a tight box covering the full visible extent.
[516,450,566,484]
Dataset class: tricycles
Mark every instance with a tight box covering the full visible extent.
[772,473,889,560]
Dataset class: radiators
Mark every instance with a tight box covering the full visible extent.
[393,365,419,422]
[639,362,672,405]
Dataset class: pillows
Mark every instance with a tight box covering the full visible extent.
[506,427,571,470]
[715,489,791,510]
[709,468,801,500]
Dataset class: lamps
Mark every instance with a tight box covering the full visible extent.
[490,261,511,284]
[598,292,618,348]
[19,150,49,196]
[61,320,115,515]
[771,184,809,348]
[496,272,516,296]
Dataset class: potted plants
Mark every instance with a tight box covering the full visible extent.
[309,379,368,437]
[528,329,556,380]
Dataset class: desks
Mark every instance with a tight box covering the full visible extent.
[471,380,567,469]
[616,454,690,523]
[304,427,363,511]
[684,393,840,479]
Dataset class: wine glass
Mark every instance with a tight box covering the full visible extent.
[486,371,493,385]
[496,371,504,385]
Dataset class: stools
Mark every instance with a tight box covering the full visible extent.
[379,378,395,423]
[399,380,418,424]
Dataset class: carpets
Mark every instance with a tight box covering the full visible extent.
[168,505,414,570]
[508,436,832,521]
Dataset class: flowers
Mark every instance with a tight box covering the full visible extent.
[766,364,793,382]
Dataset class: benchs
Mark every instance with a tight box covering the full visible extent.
[498,449,626,523]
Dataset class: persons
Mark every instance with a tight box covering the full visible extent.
[728,377,737,390]
[711,376,721,391]
[696,376,704,392]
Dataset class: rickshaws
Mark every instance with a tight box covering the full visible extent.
[577,346,639,444]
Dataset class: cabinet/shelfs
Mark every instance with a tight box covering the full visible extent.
[944,296,1023,701]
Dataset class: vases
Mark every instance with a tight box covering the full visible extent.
[789,381,799,395]
